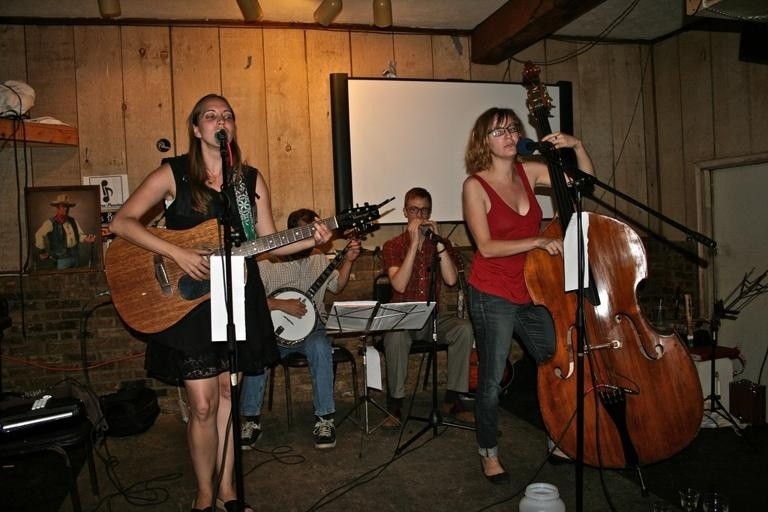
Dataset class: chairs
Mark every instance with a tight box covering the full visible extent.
[266,341,362,429]
[371,272,448,416]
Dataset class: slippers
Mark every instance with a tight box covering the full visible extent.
[191,499,213,512]
[215,498,256,512]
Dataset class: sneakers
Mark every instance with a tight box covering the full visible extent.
[240,420,265,450]
[311,414,338,450]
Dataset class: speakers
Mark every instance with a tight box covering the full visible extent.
[739,32,768,65]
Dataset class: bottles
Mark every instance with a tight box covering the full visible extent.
[517,482,566,511]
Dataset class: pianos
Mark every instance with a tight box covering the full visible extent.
[1,393,100,508]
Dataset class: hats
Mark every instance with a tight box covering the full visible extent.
[49,193,76,208]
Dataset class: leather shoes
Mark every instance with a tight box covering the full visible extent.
[442,388,480,404]
[548,451,577,466]
[479,456,510,483]
[382,404,405,430]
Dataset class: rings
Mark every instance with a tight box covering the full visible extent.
[554,136,558,140]
[553,248,558,251]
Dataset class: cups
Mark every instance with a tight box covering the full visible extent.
[647,486,730,512]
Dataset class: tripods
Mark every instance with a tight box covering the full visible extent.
[703,299,755,448]
[335,333,412,459]
[397,240,502,455]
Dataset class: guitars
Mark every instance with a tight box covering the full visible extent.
[104,203,379,333]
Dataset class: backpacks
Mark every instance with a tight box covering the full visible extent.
[98,384,161,440]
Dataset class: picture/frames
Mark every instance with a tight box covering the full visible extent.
[82,174,129,206]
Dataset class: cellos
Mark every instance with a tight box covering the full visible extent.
[522,61,704,470]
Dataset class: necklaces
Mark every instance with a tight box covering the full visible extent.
[203,167,223,185]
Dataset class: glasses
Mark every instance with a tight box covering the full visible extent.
[405,206,431,215]
[488,123,522,137]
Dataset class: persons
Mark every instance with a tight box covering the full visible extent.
[237,207,362,451]
[379,185,478,426]
[106,92,334,510]
[462,106,596,484]
[34,193,98,270]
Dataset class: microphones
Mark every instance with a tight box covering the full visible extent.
[422,226,443,244]
[516,137,553,156]
[216,129,227,141]
